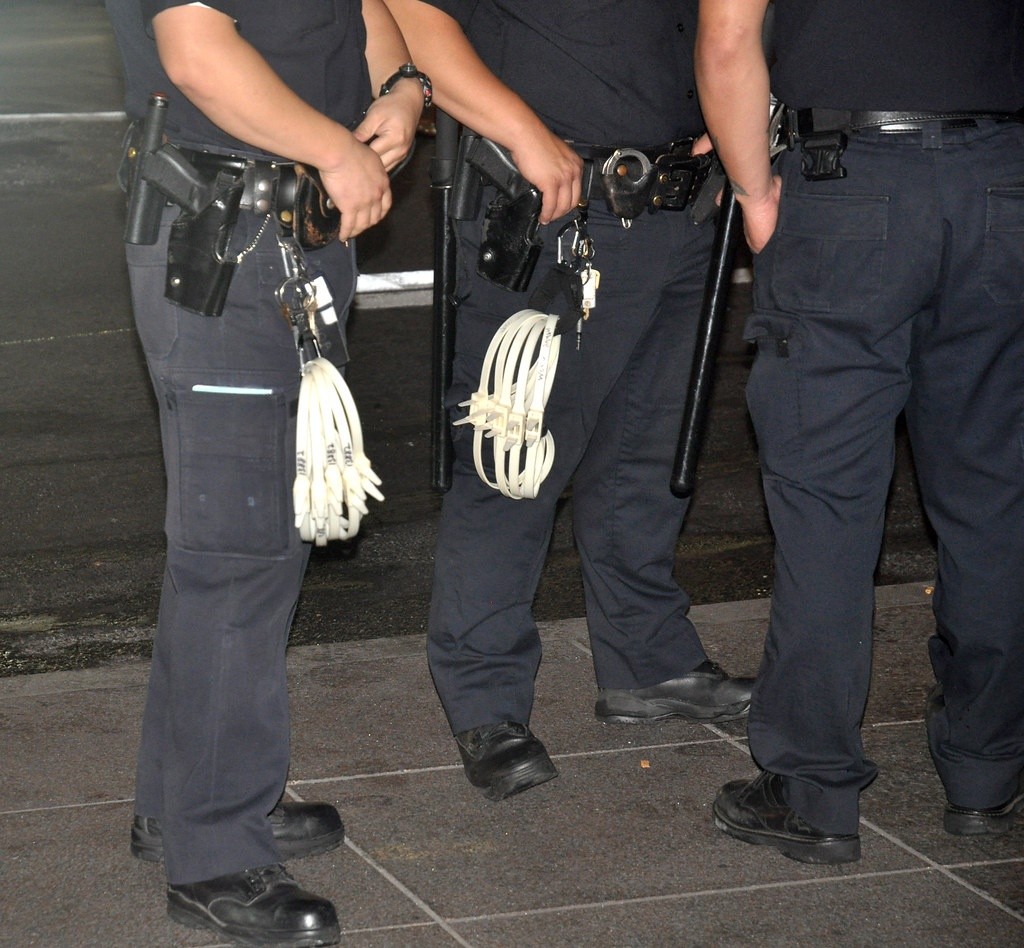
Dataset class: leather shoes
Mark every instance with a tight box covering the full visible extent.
[599,658,757,727]
[711,769,863,866]
[943,768,1024,835]
[165,862,342,947]
[130,798,344,866]
[453,718,559,802]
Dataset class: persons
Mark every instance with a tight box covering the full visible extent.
[692,0,1024,865]
[106,0,426,947]
[381,0,761,800]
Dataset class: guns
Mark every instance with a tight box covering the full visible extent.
[140,143,242,313]
[465,136,542,283]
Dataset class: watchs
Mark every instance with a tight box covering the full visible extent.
[378,62,432,109]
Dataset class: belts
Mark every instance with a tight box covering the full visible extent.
[565,136,695,164]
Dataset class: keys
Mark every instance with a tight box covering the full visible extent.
[274,232,307,279]
[583,268,601,321]
